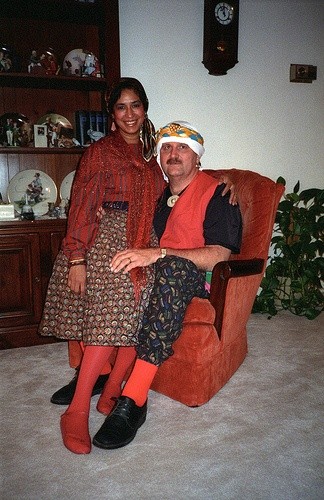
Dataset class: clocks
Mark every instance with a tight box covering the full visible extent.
[201,0,239,76]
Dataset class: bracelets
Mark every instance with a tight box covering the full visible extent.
[68,259,87,269]
[159,247,167,262]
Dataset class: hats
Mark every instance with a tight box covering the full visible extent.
[156,120,204,183]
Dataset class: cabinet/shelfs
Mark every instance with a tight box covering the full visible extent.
[0,0,120,353]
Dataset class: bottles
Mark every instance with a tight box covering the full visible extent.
[6,124,14,146]
[47,130,55,147]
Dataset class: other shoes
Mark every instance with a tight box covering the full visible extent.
[96,378,123,415]
[60,410,92,454]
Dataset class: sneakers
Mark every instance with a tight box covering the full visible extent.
[50,363,113,405]
[92,394,148,450]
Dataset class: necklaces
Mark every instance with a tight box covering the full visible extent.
[166,182,191,208]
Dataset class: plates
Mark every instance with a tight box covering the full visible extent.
[60,170,76,201]
[0,43,20,72]
[24,46,62,75]
[7,169,58,216]
[63,49,99,76]
[0,111,34,145]
[37,113,74,139]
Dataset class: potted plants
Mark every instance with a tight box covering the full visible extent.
[254,176,324,321]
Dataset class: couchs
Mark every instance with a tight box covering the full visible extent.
[69,168,286,408]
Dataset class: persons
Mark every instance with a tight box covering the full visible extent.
[37,76,243,455]
